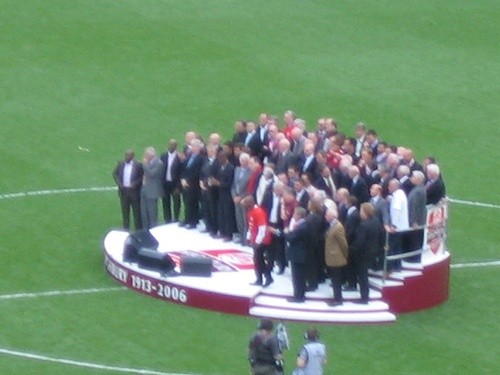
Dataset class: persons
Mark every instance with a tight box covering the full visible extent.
[291,326,328,375]
[181,109,447,307]
[138,146,164,228]
[245,319,286,375]
[158,139,184,224]
[110,150,145,231]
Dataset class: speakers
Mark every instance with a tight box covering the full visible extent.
[137,247,176,274]
[180,256,212,278]
[124,228,159,263]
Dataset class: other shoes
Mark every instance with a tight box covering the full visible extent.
[342,285,357,291]
[217,232,225,238]
[327,299,342,305]
[264,263,273,271]
[263,277,273,287]
[185,222,196,229]
[180,220,190,226]
[277,268,284,274]
[249,279,262,285]
[353,297,367,304]
[305,285,316,290]
[285,295,305,303]
[210,231,217,237]
[223,234,232,241]
[199,227,208,232]
